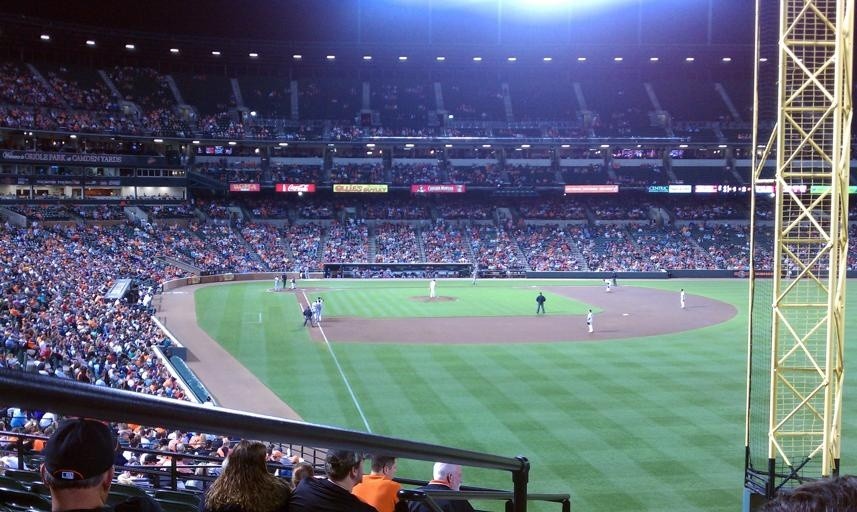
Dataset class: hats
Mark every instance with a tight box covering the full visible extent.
[42,420,113,479]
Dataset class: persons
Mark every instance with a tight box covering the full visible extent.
[282,446,378,512]
[40,420,165,512]
[199,441,292,512]
[395,462,476,512]
[758,474,857,512]
[352,455,403,512]
